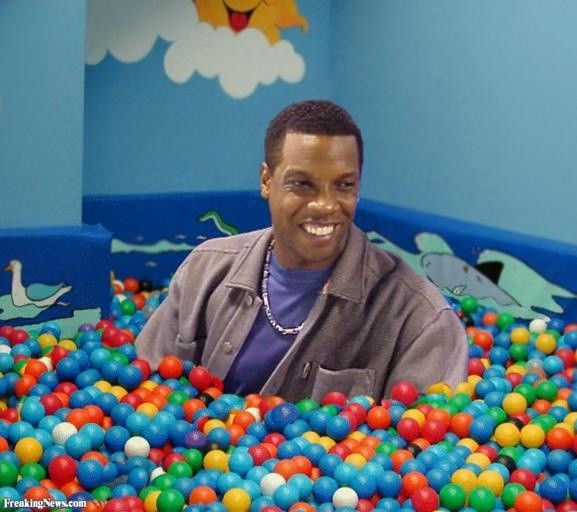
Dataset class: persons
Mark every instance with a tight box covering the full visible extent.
[131,99,469,404]
[0,276,577,512]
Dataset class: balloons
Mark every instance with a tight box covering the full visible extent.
[1,278,577,512]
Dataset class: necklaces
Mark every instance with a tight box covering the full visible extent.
[262,238,306,334]
